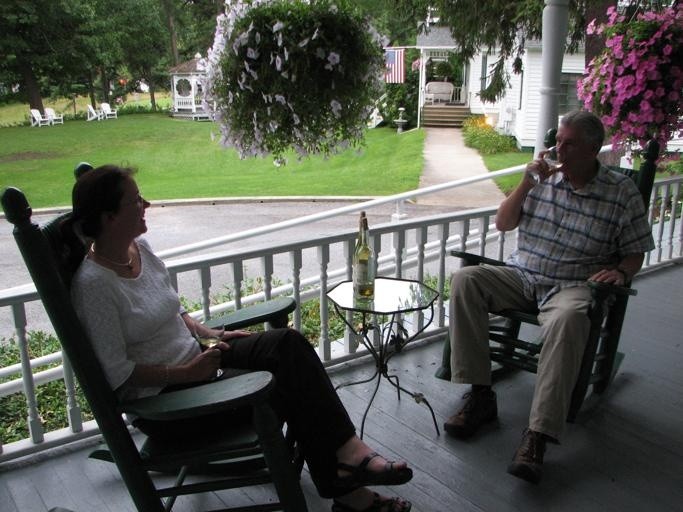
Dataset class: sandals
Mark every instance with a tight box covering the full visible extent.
[332,490,412,512]
[335,449,413,494]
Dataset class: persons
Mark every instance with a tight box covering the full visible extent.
[444,108,656,484]
[71,161,415,511]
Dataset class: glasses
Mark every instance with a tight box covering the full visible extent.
[111,190,142,215]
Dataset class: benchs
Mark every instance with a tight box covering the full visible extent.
[426,82,454,104]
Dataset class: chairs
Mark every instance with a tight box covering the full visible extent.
[0,162,308,512]
[44,108,64,126]
[101,103,118,120]
[29,109,50,128]
[86,105,104,122]
[436,128,660,420]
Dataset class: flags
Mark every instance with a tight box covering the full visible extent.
[385,49,405,83]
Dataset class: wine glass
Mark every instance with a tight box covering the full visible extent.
[526,146,568,186]
[193,314,225,378]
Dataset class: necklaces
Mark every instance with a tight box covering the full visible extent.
[91,244,136,272]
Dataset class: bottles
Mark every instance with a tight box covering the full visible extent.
[352,210,375,304]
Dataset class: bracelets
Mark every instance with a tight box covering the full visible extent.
[158,361,172,391]
[616,262,627,284]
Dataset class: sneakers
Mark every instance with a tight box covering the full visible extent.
[507,428,547,485]
[442,389,497,434]
